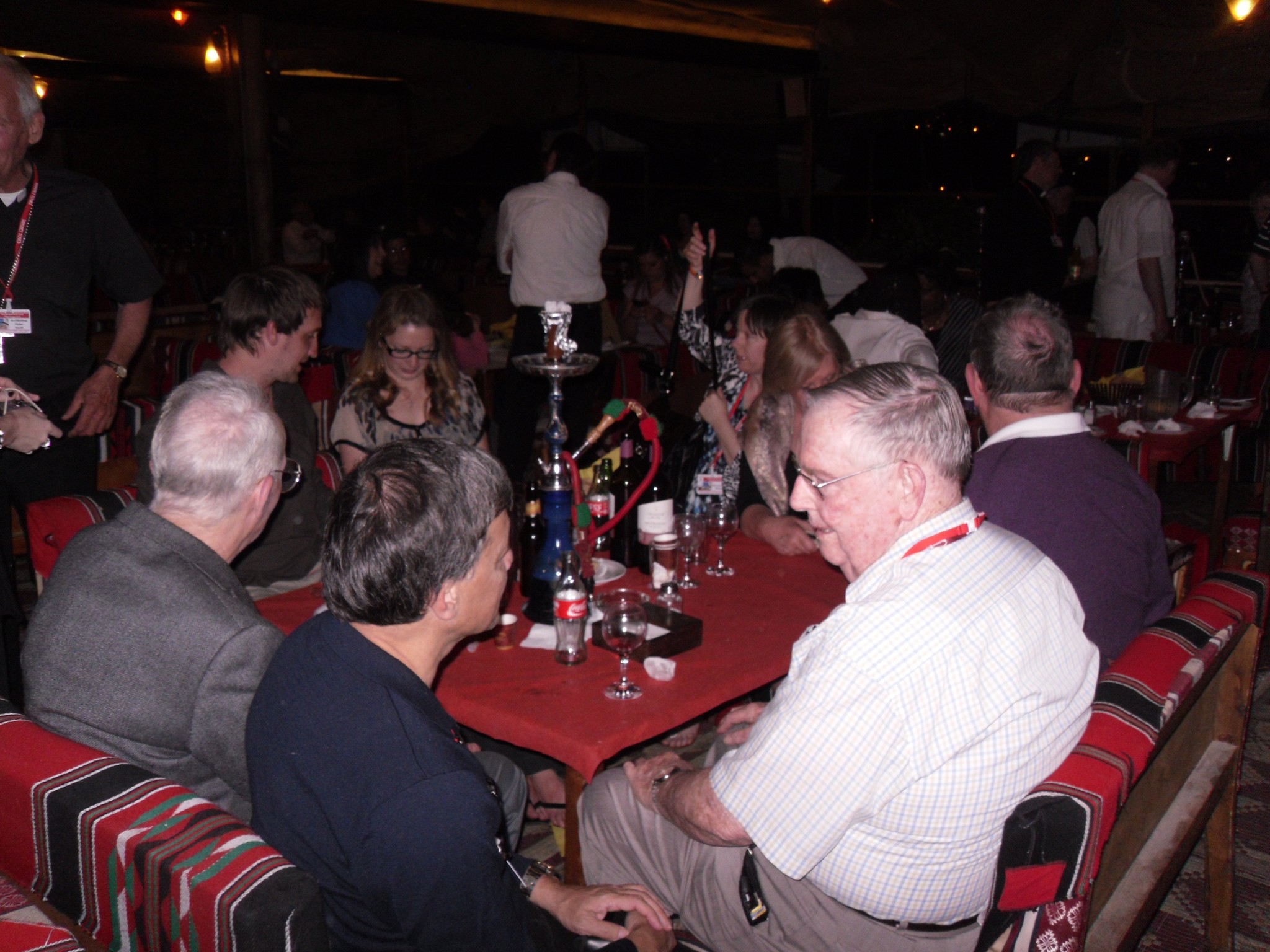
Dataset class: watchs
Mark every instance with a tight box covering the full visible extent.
[651,767,682,804]
[101,359,127,379]
[519,861,560,896]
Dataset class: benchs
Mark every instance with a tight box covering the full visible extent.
[974,570,1270,952]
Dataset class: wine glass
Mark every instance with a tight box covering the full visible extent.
[704,503,738,576]
[672,515,706,589]
[601,601,644,700]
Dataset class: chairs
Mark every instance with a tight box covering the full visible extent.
[0,336,347,952]
[1075,333,1270,483]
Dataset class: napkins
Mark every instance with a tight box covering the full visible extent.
[1219,398,1256,403]
[520,622,557,650]
[1117,421,1147,438]
[1096,405,1119,419]
[1154,418,1181,432]
[1186,402,1218,419]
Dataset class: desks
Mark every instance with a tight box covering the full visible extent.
[254,514,846,882]
[1078,403,1260,532]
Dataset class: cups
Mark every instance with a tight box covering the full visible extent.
[545,319,564,361]
[493,613,518,650]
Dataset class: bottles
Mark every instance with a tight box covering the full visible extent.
[656,582,683,614]
[1082,401,1097,426]
[552,551,589,663]
[520,431,675,599]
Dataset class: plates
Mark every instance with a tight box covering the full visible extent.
[590,557,627,586]
[1215,398,1253,411]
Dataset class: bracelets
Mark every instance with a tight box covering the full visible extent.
[689,266,702,280]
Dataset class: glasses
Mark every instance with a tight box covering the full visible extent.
[791,452,910,501]
[381,335,438,360]
[259,458,302,492]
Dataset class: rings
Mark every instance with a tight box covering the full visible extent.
[42,438,50,447]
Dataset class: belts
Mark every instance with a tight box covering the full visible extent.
[857,908,978,933]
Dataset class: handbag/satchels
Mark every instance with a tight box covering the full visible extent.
[628,400,708,506]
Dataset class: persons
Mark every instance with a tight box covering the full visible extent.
[977,137,1097,306]
[0,52,158,712]
[1241,183,1270,347]
[24,216,1174,952]
[1090,141,1178,342]
[496,129,610,469]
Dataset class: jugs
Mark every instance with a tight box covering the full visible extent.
[1143,370,1193,421]
